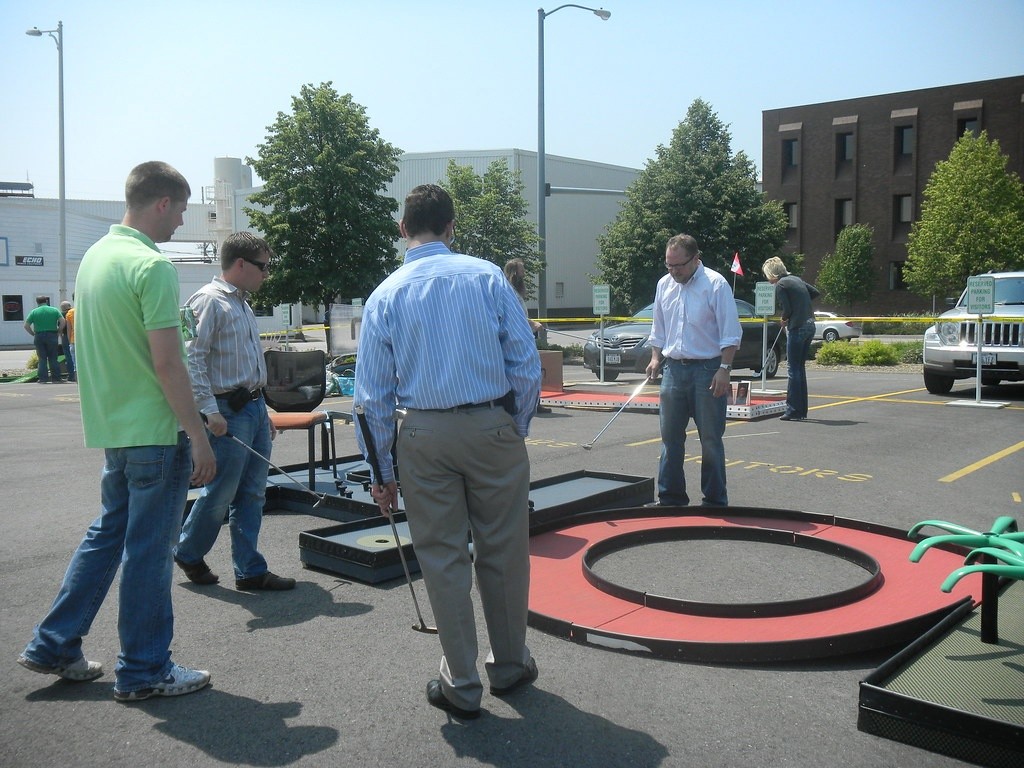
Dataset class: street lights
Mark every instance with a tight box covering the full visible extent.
[538,4,611,350]
[25,21,67,303]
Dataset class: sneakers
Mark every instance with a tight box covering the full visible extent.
[114,665,211,698]
[173,554,219,586]
[234,572,295,592]
[16,655,104,681]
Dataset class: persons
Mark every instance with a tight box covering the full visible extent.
[172,232,293,590]
[736,385,746,404]
[646,234,743,506]
[17,162,214,700]
[24,292,77,383]
[504,260,553,413]
[763,257,819,420]
[354,183,542,719]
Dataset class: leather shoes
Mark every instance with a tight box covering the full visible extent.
[427,679,481,721]
[489,657,536,695]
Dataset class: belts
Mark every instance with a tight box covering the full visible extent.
[450,398,504,410]
[213,387,262,402]
[678,358,698,365]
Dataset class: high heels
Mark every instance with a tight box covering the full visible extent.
[779,411,806,421]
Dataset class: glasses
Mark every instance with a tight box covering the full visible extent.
[236,256,271,272]
[664,254,695,270]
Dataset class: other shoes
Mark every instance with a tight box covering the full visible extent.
[38,380,46,384]
[701,501,726,506]
[644,501,690,507]
[53,378,63,383]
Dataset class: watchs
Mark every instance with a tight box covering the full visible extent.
[720,363,732,372]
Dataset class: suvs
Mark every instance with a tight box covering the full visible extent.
[923,271,1024,395]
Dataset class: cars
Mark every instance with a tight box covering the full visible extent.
[583,299,788,382]
[785,312,863,343]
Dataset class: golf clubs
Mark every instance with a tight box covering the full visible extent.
[581,355,667,451]
[353,406,438,635]
[198,410,327,509]
[751,321,783,378]
